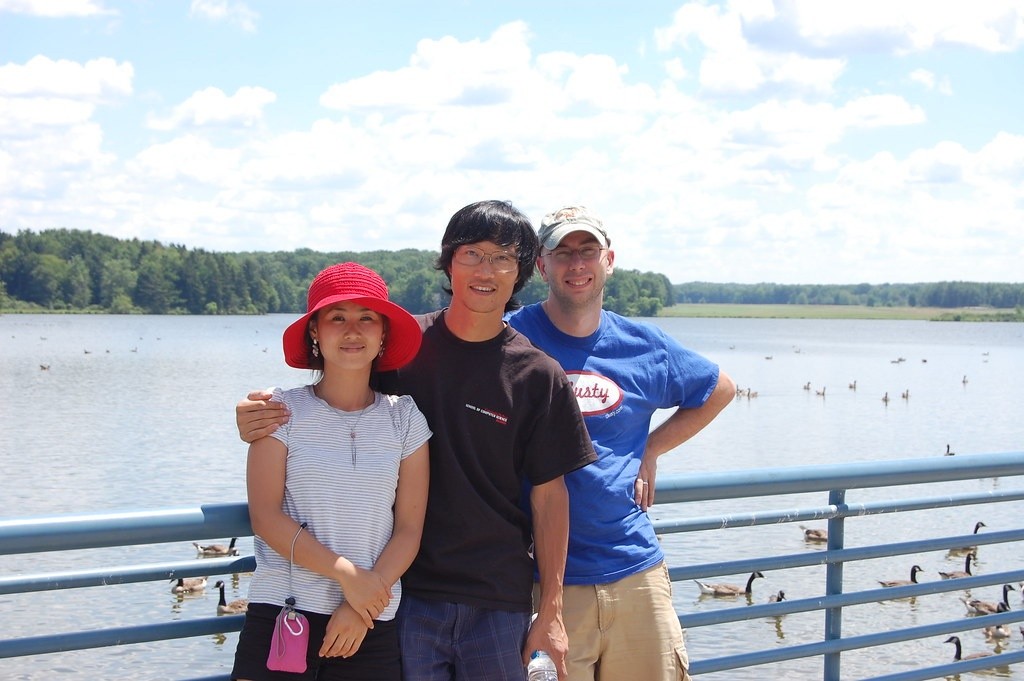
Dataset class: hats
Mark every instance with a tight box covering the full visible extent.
[283,262,421,373]
[537,203,612,251]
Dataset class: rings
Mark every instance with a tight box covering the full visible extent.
[643,481,649,486]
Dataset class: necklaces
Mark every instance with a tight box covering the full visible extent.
[314,382,375,471]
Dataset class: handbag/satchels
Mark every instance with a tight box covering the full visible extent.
[265,524,310,673]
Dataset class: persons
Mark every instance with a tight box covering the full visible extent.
[230,262,435,681]
[502,201,735,681]
[234,199,601,681]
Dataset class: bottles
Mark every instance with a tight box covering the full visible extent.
[527,612,559,680]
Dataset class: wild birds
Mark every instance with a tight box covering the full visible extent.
[816,386,826,395]
[729,345,735,349]
[891,360,899,363]
[902,389,909,398]
[765,356,773,359]
[169,576,208,593]
[132,347,138,352]
[769,591,787,602]
[962,375,968,383]
[229,548,239,557]
[938,553,977,579]
[942,636,992,661]
[735,384,747,397]
[804,382,811,389]
[694,571,767,595]
[878,565,925,587]
[882,392,890,401]
[192,537,237,557]
[849,380,856,388]
[922,359,927,362]
[84,349,90,353]
[748,388,759,397]
[797,524,828,540]
[898,358,906,361]
[944,444,955,456]
[949,522,988,550]
[211,580,249,616]
[40,365,50,370]
[960,585,1016,637]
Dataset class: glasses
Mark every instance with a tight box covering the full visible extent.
[540,244,608,260]
[453,244,522,271]
